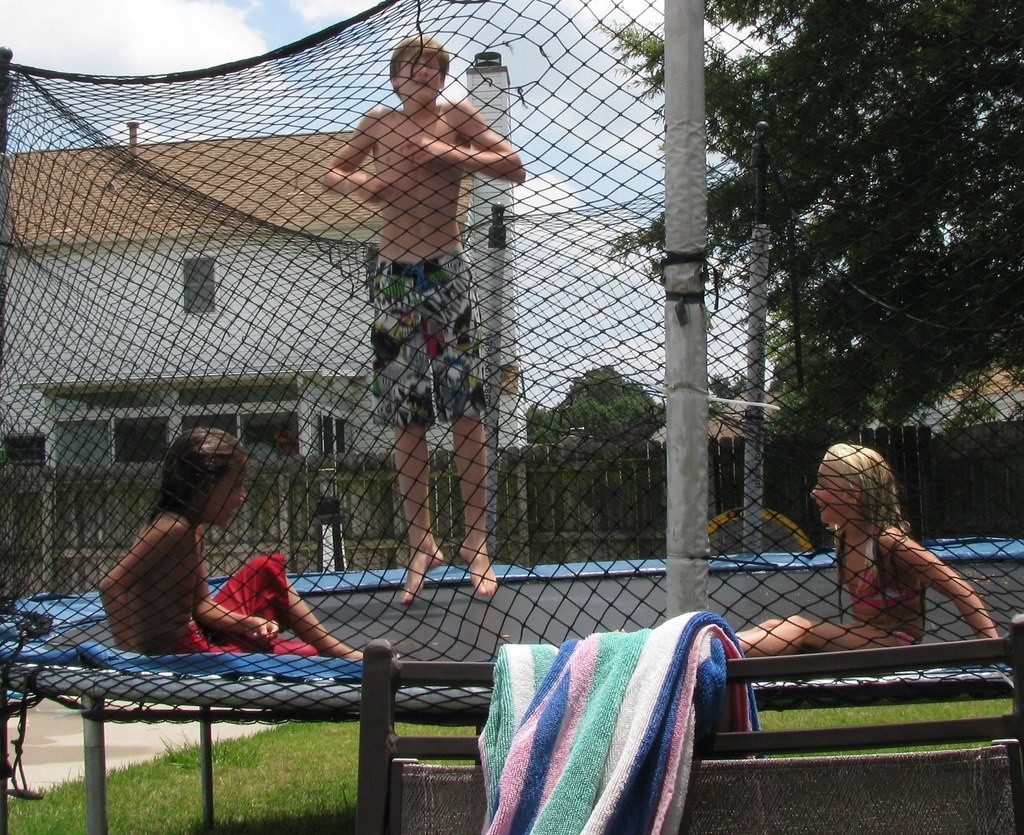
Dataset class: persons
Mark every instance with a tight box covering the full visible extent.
[96,429,366,657]
[732,443,999,660]
[320,36,528,606]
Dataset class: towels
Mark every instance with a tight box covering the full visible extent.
[478,606,762,835]
